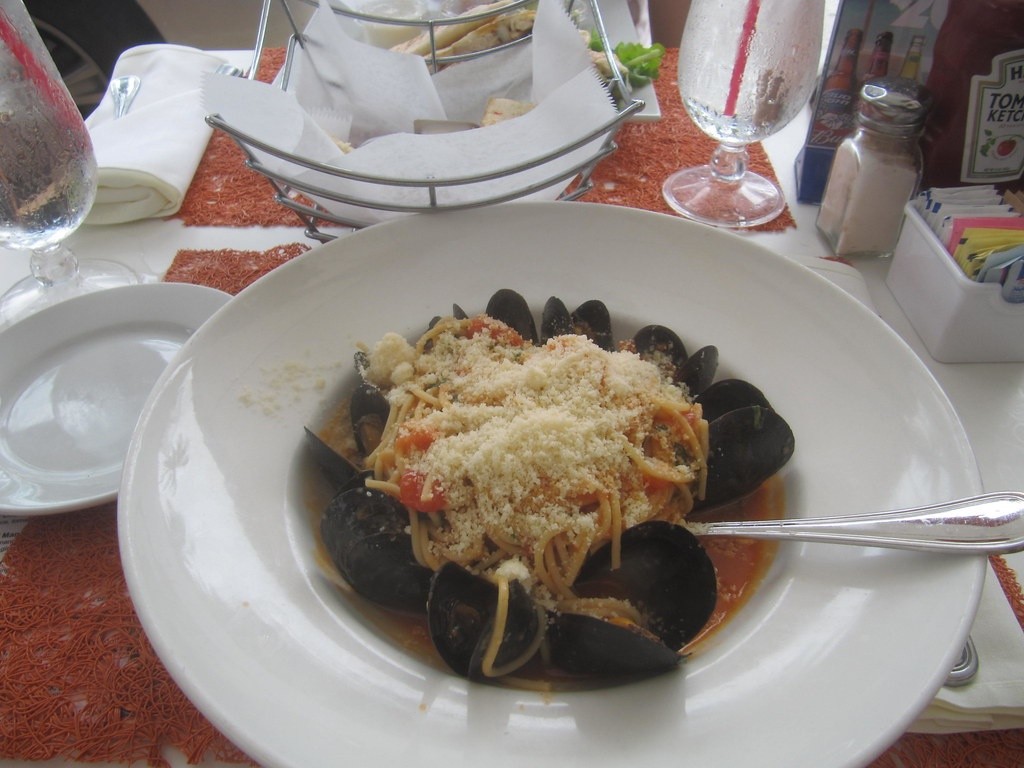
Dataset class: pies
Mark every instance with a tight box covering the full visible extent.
[389,0,536,74]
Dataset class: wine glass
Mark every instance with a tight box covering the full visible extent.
[663,0,826,227]
[0,3,140,320]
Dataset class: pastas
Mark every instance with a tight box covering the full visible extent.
[364,319,711,678]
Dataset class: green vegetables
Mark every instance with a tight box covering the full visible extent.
[589,27,666,78]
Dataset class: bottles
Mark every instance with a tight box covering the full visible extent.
[815,77,926,256]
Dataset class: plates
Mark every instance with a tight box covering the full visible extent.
[2,282,234,517]
[117,202,984,767]
